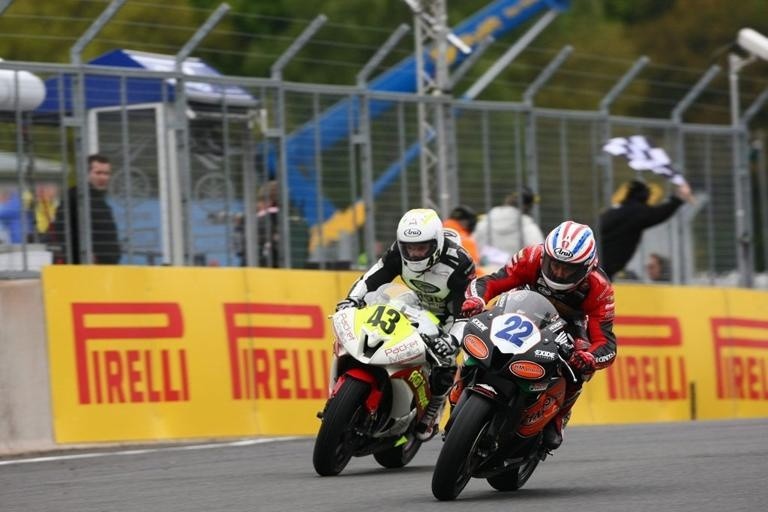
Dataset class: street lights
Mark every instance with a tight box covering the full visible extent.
[727,27,768,286]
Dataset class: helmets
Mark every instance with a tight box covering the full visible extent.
[397,208,444,272]
[542,221,597,292]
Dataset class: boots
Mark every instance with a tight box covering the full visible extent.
[543,392,581,448]
[415,395,446,440]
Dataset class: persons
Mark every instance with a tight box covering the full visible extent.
[209,184,284,266]
[472,188,544,275]
[449,219,617,446]
[44,155,122,265]
[443,206,480,270]
[645,254,671,283]
[328,209,476,441]
[596,177,691,283]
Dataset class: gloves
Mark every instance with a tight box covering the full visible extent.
[434,335,459,357]
[335,297,366,312]
[461,297,483,317]
[571,351,596,369]
